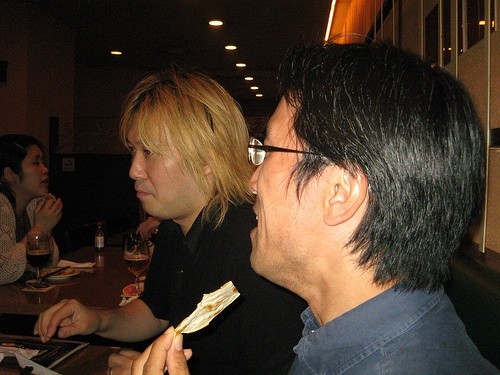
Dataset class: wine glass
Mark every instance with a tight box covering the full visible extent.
[123,233,151,298]
[25,233,54,289]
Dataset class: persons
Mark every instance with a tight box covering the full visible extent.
[130,41,500,375]
[0,133,65,286]
[136,215,161,240]
[33,68,307,375]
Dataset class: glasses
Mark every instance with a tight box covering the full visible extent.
[247,137,323,167]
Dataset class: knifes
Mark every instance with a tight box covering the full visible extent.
[42,265,70,279]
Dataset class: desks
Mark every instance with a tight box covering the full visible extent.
[0,334,132,375]
[0,229,138,314]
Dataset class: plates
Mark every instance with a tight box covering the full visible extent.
[46,272,80,282]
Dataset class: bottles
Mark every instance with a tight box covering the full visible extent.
[95,221,104,252]
